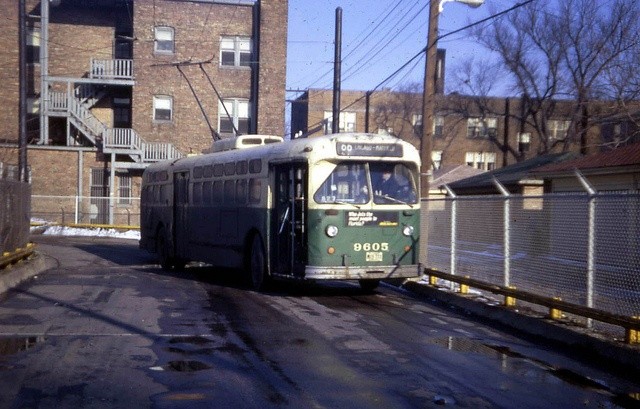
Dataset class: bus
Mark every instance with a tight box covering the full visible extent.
[138,135,424,291]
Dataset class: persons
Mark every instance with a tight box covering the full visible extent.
[376,167,400,194]
